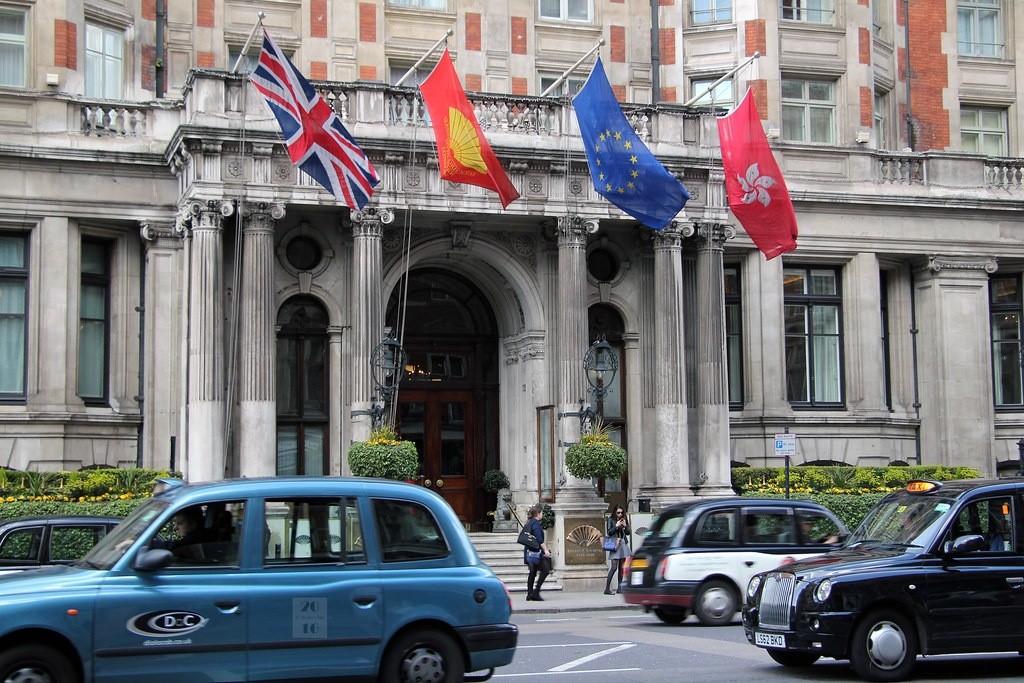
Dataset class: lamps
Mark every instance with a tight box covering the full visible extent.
[576,336,619,426]
[369,332,408,426]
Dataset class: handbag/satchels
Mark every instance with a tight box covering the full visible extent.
[600,532,621,552]
[517,521,540,552]
[526,548,541,565]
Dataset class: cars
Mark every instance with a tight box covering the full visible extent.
[0,517,168,575]
[742,477,1024,682]
[0,476,519,683]
[616,497,856,627]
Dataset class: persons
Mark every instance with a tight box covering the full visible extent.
[900,504,930,538]
[523,504,551,601]
[116,504,216,566]
[801,519,839,544]
[603,504,632,595]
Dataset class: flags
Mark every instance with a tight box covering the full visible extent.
[715,86,798,261]
[570,57,690,232]
[244,28,382,212]
[419,47,520,212]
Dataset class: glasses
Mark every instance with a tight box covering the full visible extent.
[616,512,623,514]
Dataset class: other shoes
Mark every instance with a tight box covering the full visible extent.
[616,589,622,593]
[604,591,615,595]
[526,590,543,601]
